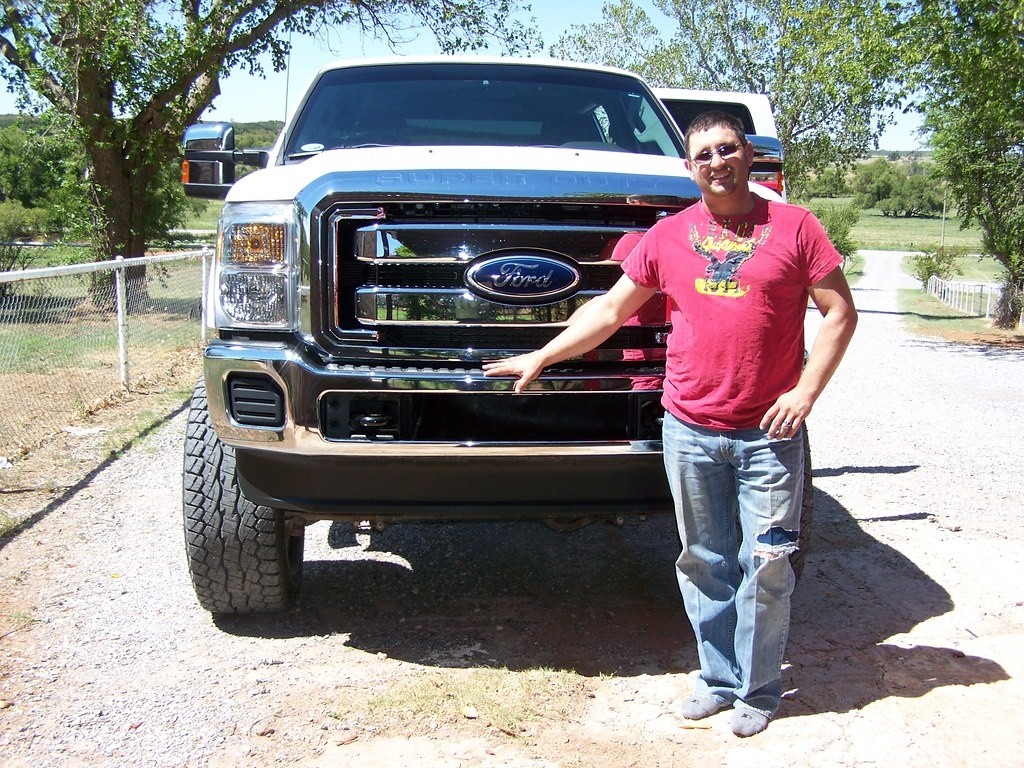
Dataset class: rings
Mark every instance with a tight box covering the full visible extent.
[783,419,793,428]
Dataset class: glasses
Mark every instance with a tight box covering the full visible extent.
[689,143,743,165]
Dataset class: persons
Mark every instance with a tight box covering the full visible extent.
[483,107,857,740]
